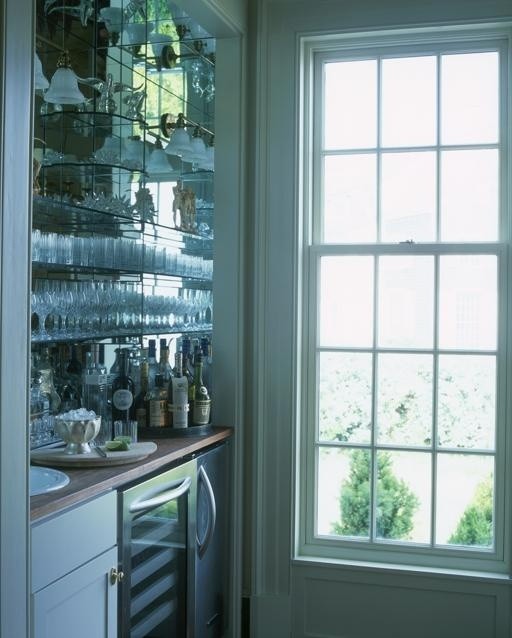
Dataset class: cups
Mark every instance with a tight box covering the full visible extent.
[32,230,213,280]
[114,420,138,443]
[31,415,57,448]
[88,420,112,447]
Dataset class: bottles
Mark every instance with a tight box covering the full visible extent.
[66,344,83,402]
[82,342,210,430]
[110,348,119,372]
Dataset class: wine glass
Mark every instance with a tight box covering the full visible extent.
[31,277,213,340]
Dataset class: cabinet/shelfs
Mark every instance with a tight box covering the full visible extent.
[120,458,197,638]
[196,440,235,637]
[32,1,234,452]
[30,488,120,636]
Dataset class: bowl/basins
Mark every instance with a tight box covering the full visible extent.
[53,418,103,454]
